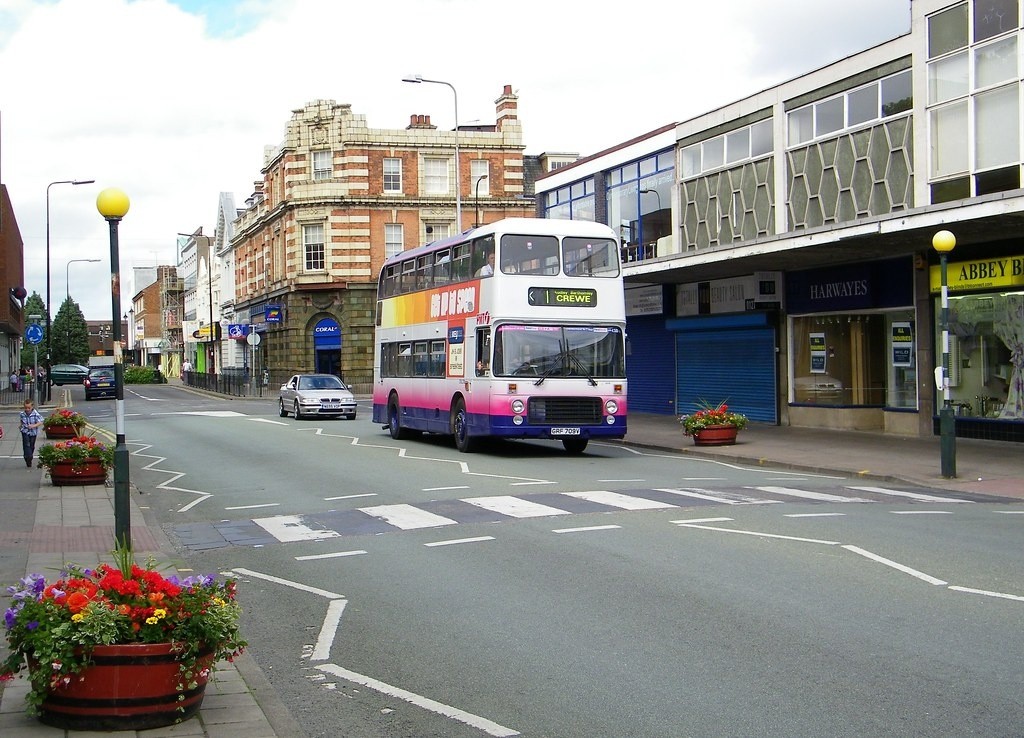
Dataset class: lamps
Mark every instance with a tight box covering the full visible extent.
[816,315,870,325]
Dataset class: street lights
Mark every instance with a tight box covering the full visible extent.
[45,178,95,401]
[66,259,100,362]
[933,228,960,479]
[640,189,664,237]
[402,75,462,233]
[177,232,217,374]
[96,184,136,554]
[475,174,488,228]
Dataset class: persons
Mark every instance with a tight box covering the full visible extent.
[182,359,192,383]
[10,365,28,391]
[18,399,44,467]
[35,362,43,374]
[477,343,534,372]
[481,253,495,277]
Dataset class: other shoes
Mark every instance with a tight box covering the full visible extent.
[27,461,32,467]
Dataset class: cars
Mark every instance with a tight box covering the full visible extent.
[84,368,116,400]
[46,363,90,386]
[278,372,357,421]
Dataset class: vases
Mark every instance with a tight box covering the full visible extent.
[23,643,215,730]
[50,457,107,486]
[44,424,81,439]
[692,425,739,445]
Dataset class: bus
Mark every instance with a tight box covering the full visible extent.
[372,221,629,454]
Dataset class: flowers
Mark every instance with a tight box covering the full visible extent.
[0,531,247,729]
[677,396,750,437]
[36,423,115,488]
[43,405,87,428]
